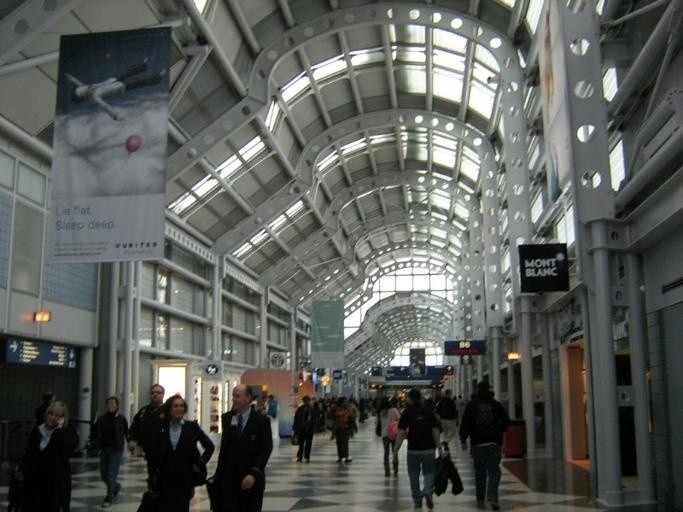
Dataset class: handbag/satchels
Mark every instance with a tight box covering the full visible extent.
[389,416,400,439]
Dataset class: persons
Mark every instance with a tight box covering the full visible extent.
[210,384,274,511]
[126,384,169,492]
[88,395,134,509]
[459,378,510,511]
[392,387,444,510]
[10,391,79,512]
[291,384,479,477]
[251,391,282,419]
[137,393,215,511]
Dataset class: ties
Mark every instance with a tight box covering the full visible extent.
[238,414,243,433]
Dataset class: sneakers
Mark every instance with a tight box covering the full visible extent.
[102,482,121,508]
[477,501,501,511]
[415,488,433,509]
[336,458,352,463]
